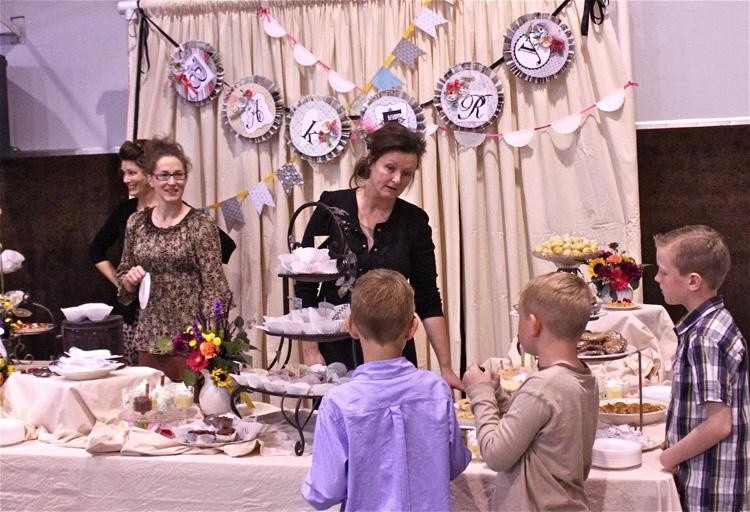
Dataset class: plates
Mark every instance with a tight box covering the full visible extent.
[639,385,672,399]
[139,272,151,311]
[572,344,639,360]
[47,361,126,380]
[510,306,608,322]
[590,398,673,471]
[601,302,642,310]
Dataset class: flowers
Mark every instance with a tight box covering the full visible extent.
[585,238,658,301]
[153,288,258,409]
[0,291,36,385]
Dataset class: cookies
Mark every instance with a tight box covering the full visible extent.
[577,330,627,355]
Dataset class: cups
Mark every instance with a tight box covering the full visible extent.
[589,365,628,400]
[121,381,195,422]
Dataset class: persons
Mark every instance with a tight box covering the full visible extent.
[116,133,237,382]
[294,119,466,410]
[653,225,750,512]
[87,139,237,366]
[299,268,472,512]
[461,270,599,512]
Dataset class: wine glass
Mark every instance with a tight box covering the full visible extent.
[529,248,605,275]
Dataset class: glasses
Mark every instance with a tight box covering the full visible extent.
[153,172,187,181]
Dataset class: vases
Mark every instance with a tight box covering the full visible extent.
[599,280,634,306]
[198,365,235,418]
